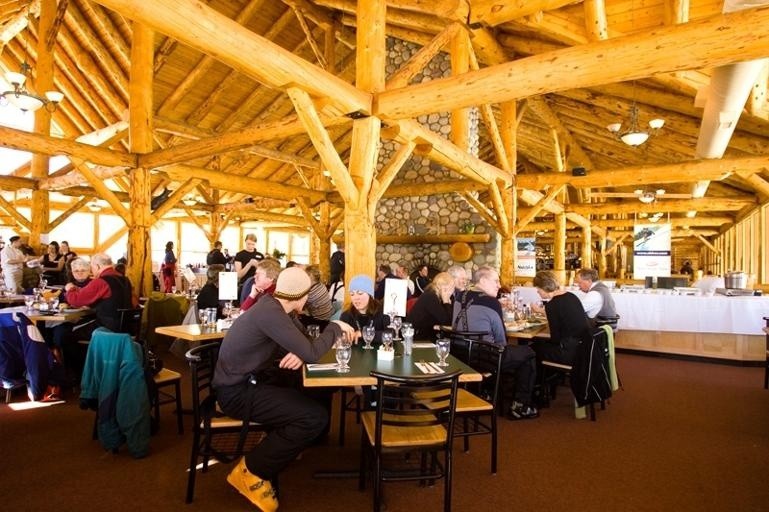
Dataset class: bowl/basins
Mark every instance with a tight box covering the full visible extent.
[609,288,715,297]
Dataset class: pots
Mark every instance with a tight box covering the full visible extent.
[724,269,747,290]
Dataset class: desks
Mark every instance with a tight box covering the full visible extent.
[302,339,482,479]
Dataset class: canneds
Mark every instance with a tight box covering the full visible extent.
[206,307,217,328]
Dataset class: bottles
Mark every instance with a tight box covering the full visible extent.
[403,323,414,355]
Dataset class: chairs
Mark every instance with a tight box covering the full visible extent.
[505,292,625,422]
[358,368,465,512]
[96,268,505,435]
[0,249,96,404]
[422,338,505,488]
[184,341,280,505]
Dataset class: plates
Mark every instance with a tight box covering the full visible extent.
[503,320,547,332]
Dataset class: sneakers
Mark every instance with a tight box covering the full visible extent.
[224,455,279,512]
[509,401,539,420]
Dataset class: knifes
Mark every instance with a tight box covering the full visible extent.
[426,360,440,373]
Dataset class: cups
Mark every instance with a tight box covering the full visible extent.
[206,308,218,329]
[645,276,653,289]
[307,324,319,338]
[171,285,196,297]
[382,332,395,345]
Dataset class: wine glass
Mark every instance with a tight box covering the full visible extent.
[335,342,353,372]
[436,339,450,369]
[224,302,232,321]
[198,310,209,329]
[362,326,376,350]
[22,278,60,315]
[391,317,402,343]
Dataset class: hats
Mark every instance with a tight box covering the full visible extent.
[348,274,375,299]
[273,266,312,300]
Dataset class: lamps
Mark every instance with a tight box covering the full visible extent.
[606,78,666,149]
[2,3,65,116]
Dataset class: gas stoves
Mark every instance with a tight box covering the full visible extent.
[715,287,752,297]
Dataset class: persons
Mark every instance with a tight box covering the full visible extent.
[680,261,713,281]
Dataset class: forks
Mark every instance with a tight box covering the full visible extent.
[419,359,431,373]
